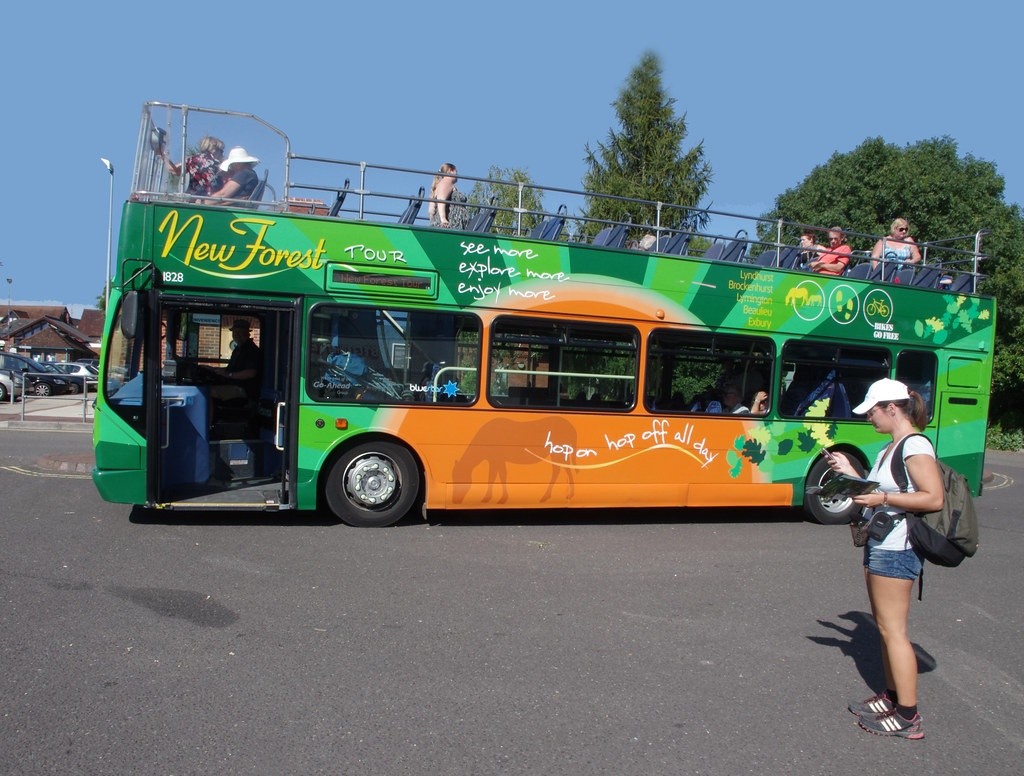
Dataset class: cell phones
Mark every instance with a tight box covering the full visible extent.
[822,447,837,464]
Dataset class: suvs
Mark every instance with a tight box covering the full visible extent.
[0,350,71,397]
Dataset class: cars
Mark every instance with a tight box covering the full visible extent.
[0,368,34,401]
[39,358,141,395]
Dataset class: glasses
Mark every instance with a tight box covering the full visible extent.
[869,407,885,415]
[899,228,909,232]
[829,237,838,241]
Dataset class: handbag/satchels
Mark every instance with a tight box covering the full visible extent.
[868,512,894,541]
[851,521,868,547]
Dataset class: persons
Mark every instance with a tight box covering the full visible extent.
[191,320,261,415]
[871,219,921,272]
[799,228,850,276]
[166,137,261,209]
[826,378,944,739]
[718,383,770,416]
[884,493,888,504]
[429,164,467,231]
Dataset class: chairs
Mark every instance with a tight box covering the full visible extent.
[952,274,980,293]
[869,251,899,281]
[893,262,916,285]
[700,234,726,260]
[592,219,614,245]
[406,186,425,224]
[540,204,568,240]
[753,240,777,266]
[720,229,749,262]
[773,237,801,268]
[530,212,551,238]
[648,226,672,252]
[603,213,632,247]
[665,220,693,254]
[477,196,499,232]
[327,178,350,217]
[399,194,415,223]
[468,203,487,230]
[244,169,269,208]
[912,258,943,286]
[848,262,872,279]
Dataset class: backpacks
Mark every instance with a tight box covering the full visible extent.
[891,433,979,567]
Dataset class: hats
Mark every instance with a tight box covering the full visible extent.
[852,378,910,415]
[230,319,253,332]
[220,148,259,171]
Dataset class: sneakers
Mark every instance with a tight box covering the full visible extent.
[848,688,897,716]
[858,708,924,739]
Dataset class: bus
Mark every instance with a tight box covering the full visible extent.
[89,100,998,527]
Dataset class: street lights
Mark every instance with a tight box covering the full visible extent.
[99,156,115,314]
[6,278,12,353]
